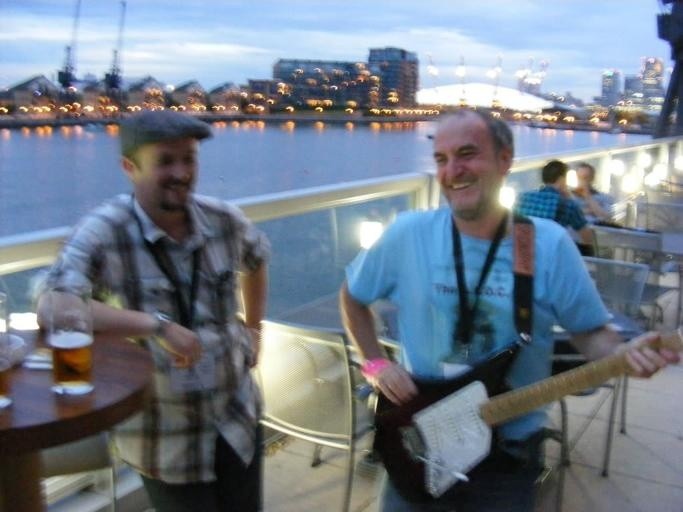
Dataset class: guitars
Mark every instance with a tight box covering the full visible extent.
[374,326,683,509]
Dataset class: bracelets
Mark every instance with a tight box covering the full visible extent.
[361,357,389,377]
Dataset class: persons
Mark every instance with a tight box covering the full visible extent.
[35,106,273,511]
[572,161,615,226]
[514,156,591,255]
[335,108,680,512]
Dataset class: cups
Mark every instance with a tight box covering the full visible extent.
[50,285,94,394]
[0,333,13,409]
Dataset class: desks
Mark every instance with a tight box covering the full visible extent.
[0,329,157,512]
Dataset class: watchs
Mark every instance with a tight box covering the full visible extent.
[151,307,174,339]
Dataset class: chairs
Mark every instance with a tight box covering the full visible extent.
[590,175,683,330]
[545,256,648,481]
[258,317,378,511]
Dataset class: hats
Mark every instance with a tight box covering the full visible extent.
[120,111,211,150]
